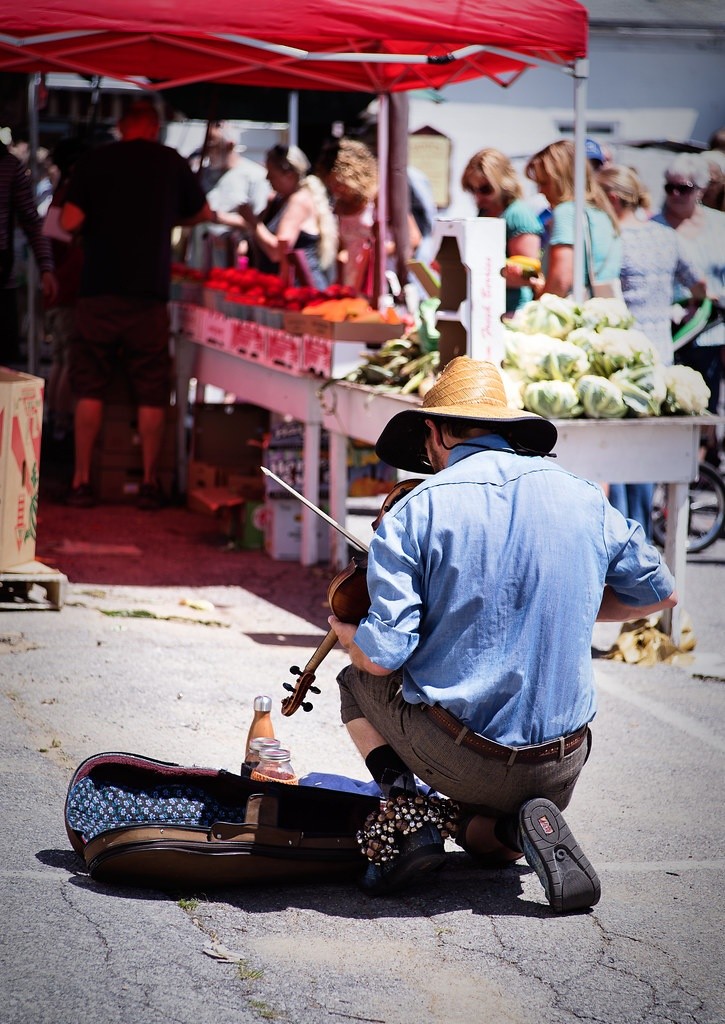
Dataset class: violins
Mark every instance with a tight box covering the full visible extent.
[279,475,427,718]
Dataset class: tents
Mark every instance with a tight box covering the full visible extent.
[0,0,590,316]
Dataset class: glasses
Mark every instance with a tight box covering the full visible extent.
[467,185,494,195]
[419,443,433,467]
[664,182,696,196]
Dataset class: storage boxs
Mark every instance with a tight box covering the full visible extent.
[171,303,406,379]
[92,398,177,498]
[186,463,329,563]
[0,365,45,572]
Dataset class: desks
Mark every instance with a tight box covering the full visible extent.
[323,379,719,657]
[171,335,325,569]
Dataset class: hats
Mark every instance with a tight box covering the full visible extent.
[374,355,558,475]
[584,138,604,166]
[120,91,164,121]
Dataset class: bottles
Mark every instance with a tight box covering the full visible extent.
[245,737,280,768]
[253,748,295,780]
[245,696,274,762]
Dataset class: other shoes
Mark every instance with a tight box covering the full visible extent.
[136,482,168,510]
[355,823,447,897]
[518,797,601,912]
[55,481,96,507]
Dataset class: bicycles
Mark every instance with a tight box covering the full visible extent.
[650,462,725,553]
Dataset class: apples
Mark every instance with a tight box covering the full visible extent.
[172,262,356,310]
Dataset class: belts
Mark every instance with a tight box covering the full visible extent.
[423,703,588,764]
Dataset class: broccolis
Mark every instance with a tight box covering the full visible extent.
[493,294,710,419]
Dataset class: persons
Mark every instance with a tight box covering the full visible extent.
[327,355,679,914]
[0,91,725,536]
